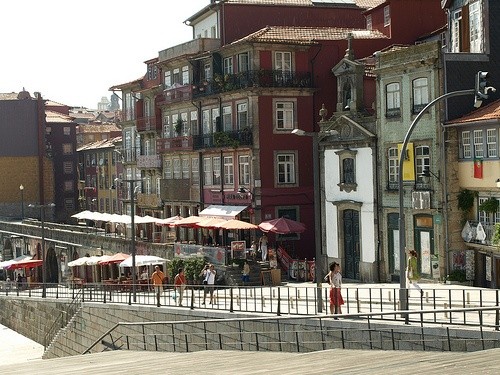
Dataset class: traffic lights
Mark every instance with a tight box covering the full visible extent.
[475,71,492,99]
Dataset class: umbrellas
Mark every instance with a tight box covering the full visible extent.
[67,253,168,280]
[71,210,306,246]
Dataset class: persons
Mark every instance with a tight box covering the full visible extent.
[324,262,344,314]
[17,274,22,287]
[259,233,269,262]
[116,223,124,236]
[405,247,424,297]
[150,266,164,306]
[174,268,186,307]
[250,240,259,260]
[241,261,250,286]
[200,263,216,305]
[206,233,212,245]
[113,271,148,292]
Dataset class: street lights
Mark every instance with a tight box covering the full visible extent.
[114,177,149,303]
[291,129,339,313]
[28,203,56,298]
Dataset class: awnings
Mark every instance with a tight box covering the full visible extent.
[199,205,248,219]
[0,255,42,270]
